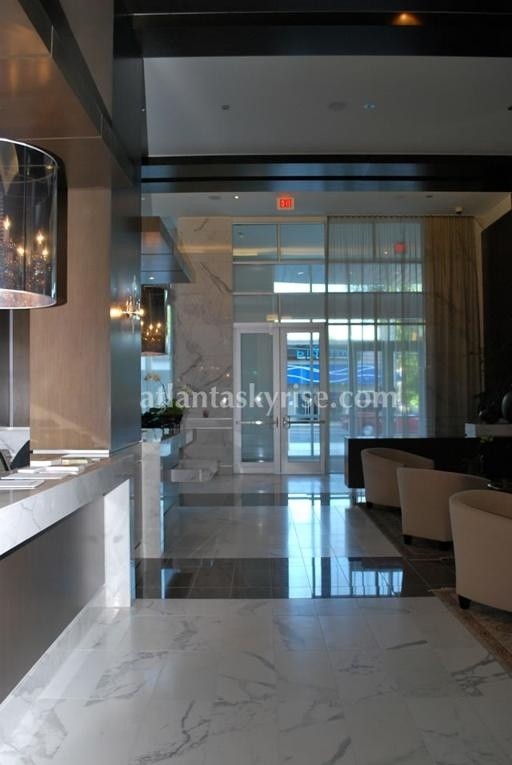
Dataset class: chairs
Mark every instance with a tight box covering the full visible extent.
[360,447,434,513]
[448,490,512,613]
[396,468,491,550]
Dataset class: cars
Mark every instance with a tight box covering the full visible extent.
[341,411,419,436]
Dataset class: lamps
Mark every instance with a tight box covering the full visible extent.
[0,137,58,311]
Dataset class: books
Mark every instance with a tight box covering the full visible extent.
[1,454,90,490]
